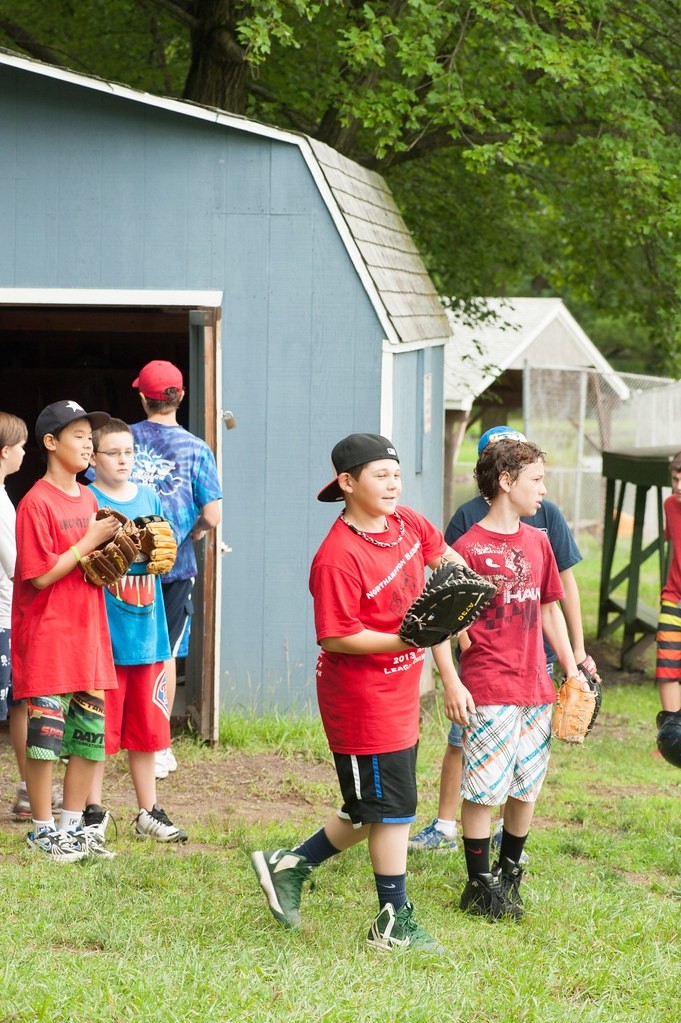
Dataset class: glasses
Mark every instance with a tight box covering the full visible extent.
[94,447,134,456]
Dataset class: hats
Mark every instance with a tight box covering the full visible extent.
[316,433,399,503]
[35,400,111,440]
[478,426,525,455]
[132,361,183,400]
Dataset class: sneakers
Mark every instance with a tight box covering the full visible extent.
[80,804,112,843]
[26,826,83,863]
[457,867,524,925]
[492,823,529,864]
[130,803,188,844]
[406,818,458,853]
[153,747,178,779]
[250,848,320,929]
[491,856,525,893]
[67,826,116,858]
[366,899,452,957]
[12,786,63,815]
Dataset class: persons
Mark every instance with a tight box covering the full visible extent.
[85,420,189,844]
[431,435,591,926]
[0,411,64,817]
[249,433,468,956]
[11,402,122,866]
[404,427,601,865]
[656,448,681,769]
[126,360,223,778]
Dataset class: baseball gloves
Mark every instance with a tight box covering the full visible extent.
[553,655,603,744]
[133,515,178,575]
[397,559,498,647]
[75,508,141,587]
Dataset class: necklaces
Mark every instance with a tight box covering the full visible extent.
[341,509,403,548]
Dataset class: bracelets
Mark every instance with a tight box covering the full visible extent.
[69,546,81,562]
